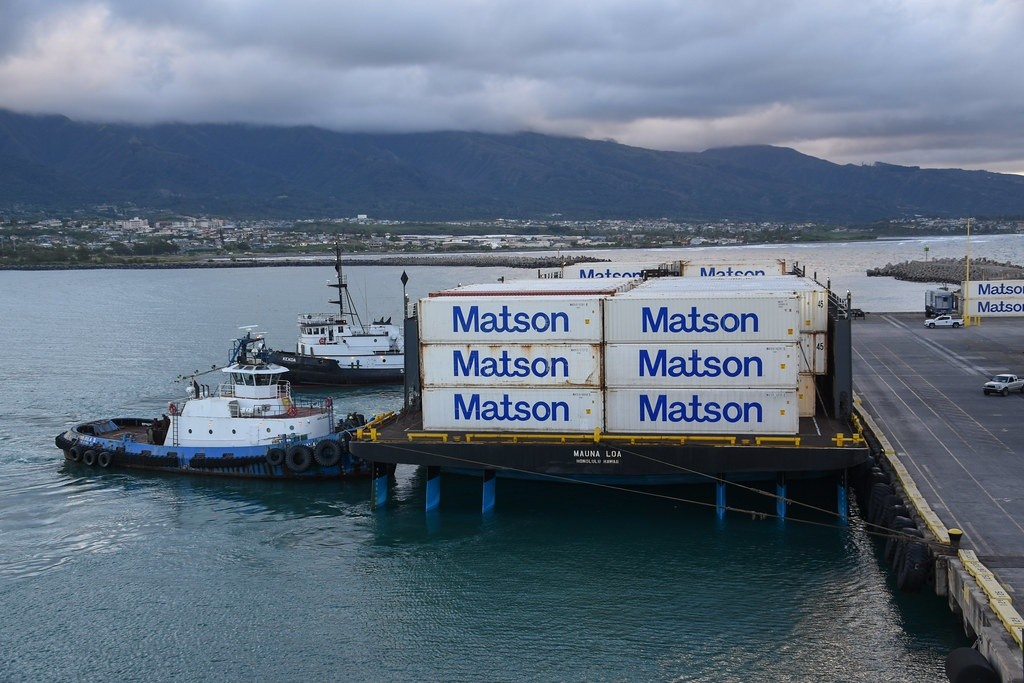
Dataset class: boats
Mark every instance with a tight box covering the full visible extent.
[247,242,404,386]
[54,324,398,479]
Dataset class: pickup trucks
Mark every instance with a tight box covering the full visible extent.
[923,315,964,329]
[981,373,1024,396]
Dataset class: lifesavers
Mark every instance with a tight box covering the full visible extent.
[69,446,83,462]
[169,405,177,414]
[84,450,98,466]
[99,452,111,467]
[288,444,312,472]
[266,448,284,465]
[319,338,324,345]
[853,455,927,592]
[315,439,342,468]
[288,407,297,417]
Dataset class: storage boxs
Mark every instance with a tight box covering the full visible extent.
[416,257,830,437]
[957,279,1024,318]
